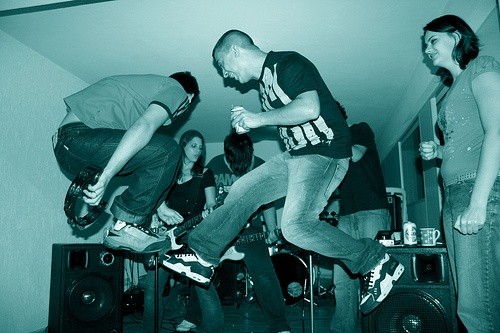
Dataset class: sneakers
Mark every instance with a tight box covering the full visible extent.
[158,253,214,287]
[103,223,170,254]
[358,252,405,315]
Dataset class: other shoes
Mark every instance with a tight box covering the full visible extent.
[175,319,197,332]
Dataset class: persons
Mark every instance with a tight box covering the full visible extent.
[204,127,295,333]
[286,97,391,332]
[412,13,500,333]
[137,130,226,333]
[54,70,201,253]
[154,29,405,316]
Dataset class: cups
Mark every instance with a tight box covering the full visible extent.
[391,232,401,241]
[420,228,440,245]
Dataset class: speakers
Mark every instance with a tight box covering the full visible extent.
[48,243,123,333]
[364,242,458,333]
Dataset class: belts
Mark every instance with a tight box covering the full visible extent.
[443,171,477,190]
[52,122,88,142]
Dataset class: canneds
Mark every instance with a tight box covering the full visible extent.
[231,106,250,135]
[403,221,417,245]
